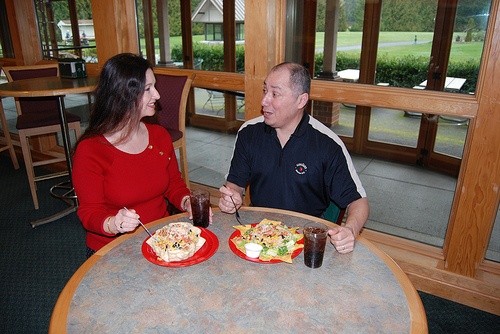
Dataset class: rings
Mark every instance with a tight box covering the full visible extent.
[120,222,123,228]
[342,246,344,249]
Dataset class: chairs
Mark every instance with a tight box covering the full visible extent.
[0,62,81,209]
[0,100,19,170]
[203,91,225,112]
[139,66,194,191]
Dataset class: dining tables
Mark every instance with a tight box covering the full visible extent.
[47,206,429,334]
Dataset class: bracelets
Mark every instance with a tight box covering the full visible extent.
[184,197,189,210]
[107,217,113,234]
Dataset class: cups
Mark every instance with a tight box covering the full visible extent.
[189,190,210,228]
[245,243,263,258]
[303,221,328,268]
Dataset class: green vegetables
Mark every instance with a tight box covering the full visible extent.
[241,230,297,255]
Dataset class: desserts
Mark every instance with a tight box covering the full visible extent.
[147,221,196,263]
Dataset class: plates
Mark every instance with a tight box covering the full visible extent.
[141,225,219,267]
[229,223,305,264]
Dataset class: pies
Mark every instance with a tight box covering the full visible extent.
[230,219,305,264]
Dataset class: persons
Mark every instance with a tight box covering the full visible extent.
[70,52,215,257]
[219,61,370,254]
[65,30,70,38]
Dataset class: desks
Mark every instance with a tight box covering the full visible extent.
[0,75,96,227]
[420,77,466,91]
[337,69,360,84]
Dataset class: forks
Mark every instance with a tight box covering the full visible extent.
[223,185,246,227]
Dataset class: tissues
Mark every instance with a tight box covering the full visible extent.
[56,52,87,79]
